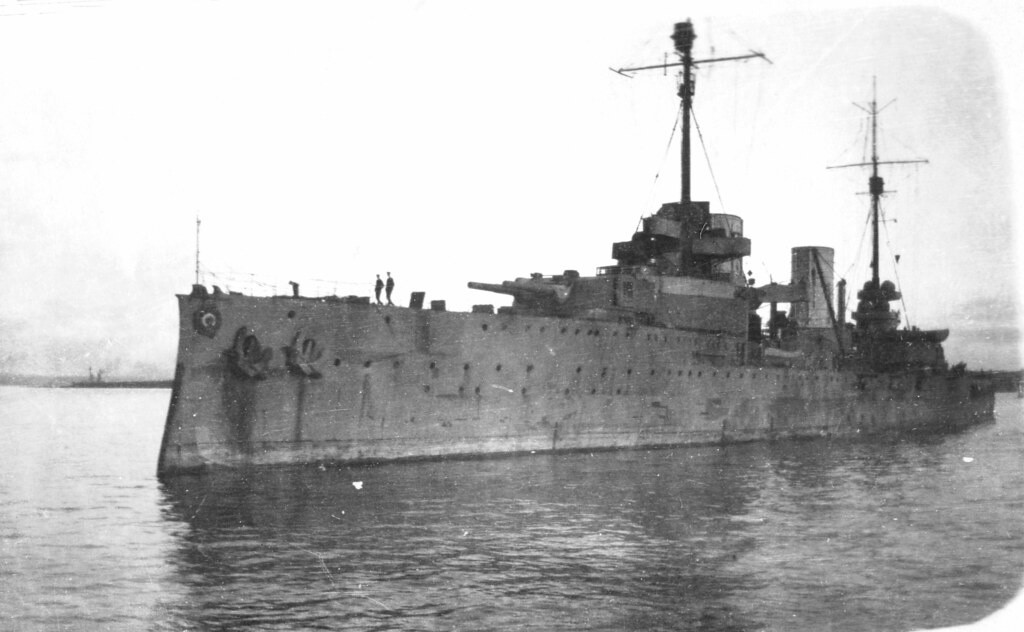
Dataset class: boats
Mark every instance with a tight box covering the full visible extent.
[152,16,998,475]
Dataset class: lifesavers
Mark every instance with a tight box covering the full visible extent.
[193,305,223,336]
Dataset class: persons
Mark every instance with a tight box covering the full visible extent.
[289,280,299,296]
[386,272,394,305]
[375,274,384,305]
